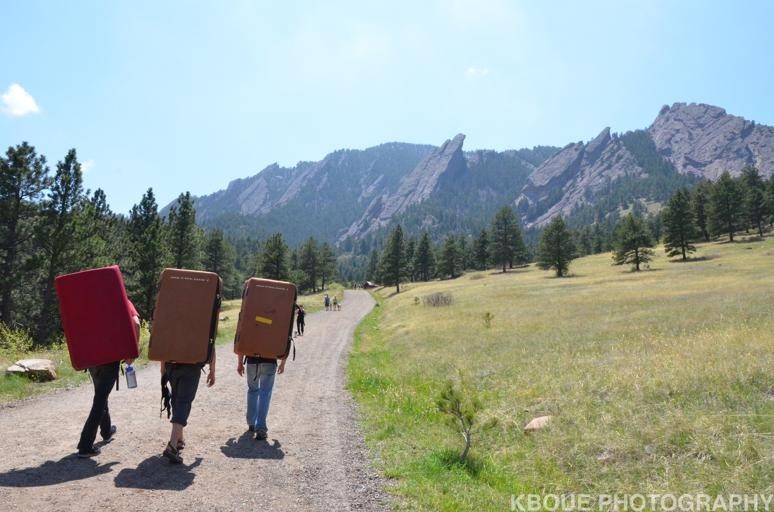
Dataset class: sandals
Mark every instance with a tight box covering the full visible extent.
[163,440,185,464]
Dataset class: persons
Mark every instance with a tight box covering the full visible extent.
[237,355,286,440]
[325,294,341,311]
[161,343,215,464]
[295,305,305,336]
[78,299,140,458]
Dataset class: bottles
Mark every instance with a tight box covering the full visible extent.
[125,365,137,388]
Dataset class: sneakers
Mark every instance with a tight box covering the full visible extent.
[103,425,117,442]
[78,447,101,457]
[249,425,268,440]
